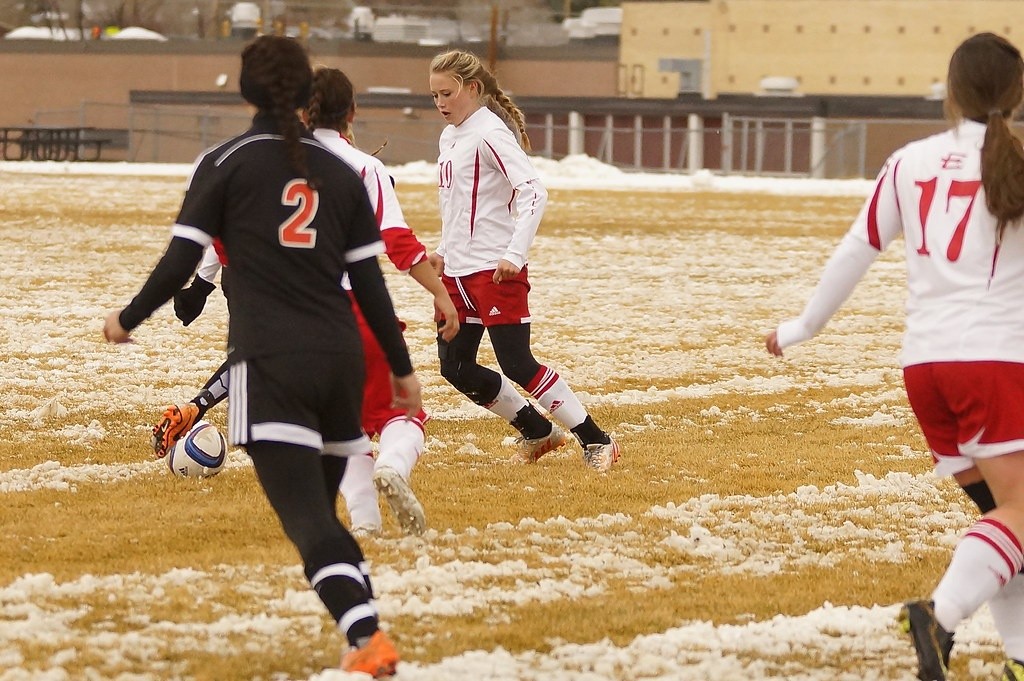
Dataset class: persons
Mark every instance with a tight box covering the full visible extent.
[429,51,621,471]
[151,66,459,538]
[103,37,423,680]
[768,33,1024,681]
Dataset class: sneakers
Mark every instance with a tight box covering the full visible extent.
[897,600,955,681]
[340,629,401,677]
[151,403,199,458]
[1001,658,1024,681]
[582,430,622,475]
[513,420,566,462]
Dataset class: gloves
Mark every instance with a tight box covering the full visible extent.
[172,273,217,326]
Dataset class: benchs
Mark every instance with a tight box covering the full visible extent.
[0,138,112,161]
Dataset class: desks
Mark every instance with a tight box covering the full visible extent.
[0,126,97,160]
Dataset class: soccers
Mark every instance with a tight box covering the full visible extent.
[164,419,228,479]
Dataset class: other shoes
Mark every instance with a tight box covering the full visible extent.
[348,522,383,539]
[371,463,426,537]
